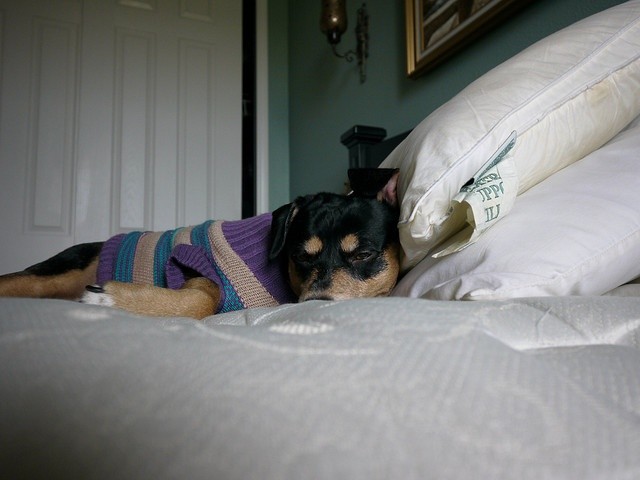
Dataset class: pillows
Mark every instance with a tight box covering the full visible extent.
[371,0,640,270]
[389,118,640,300]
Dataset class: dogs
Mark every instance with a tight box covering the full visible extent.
[0,163,409,322]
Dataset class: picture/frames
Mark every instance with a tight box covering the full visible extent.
[399,0,537,79]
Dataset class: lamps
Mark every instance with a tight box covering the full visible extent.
[320,0,367,84]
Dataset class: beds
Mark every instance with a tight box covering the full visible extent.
[1,126,638,480]
